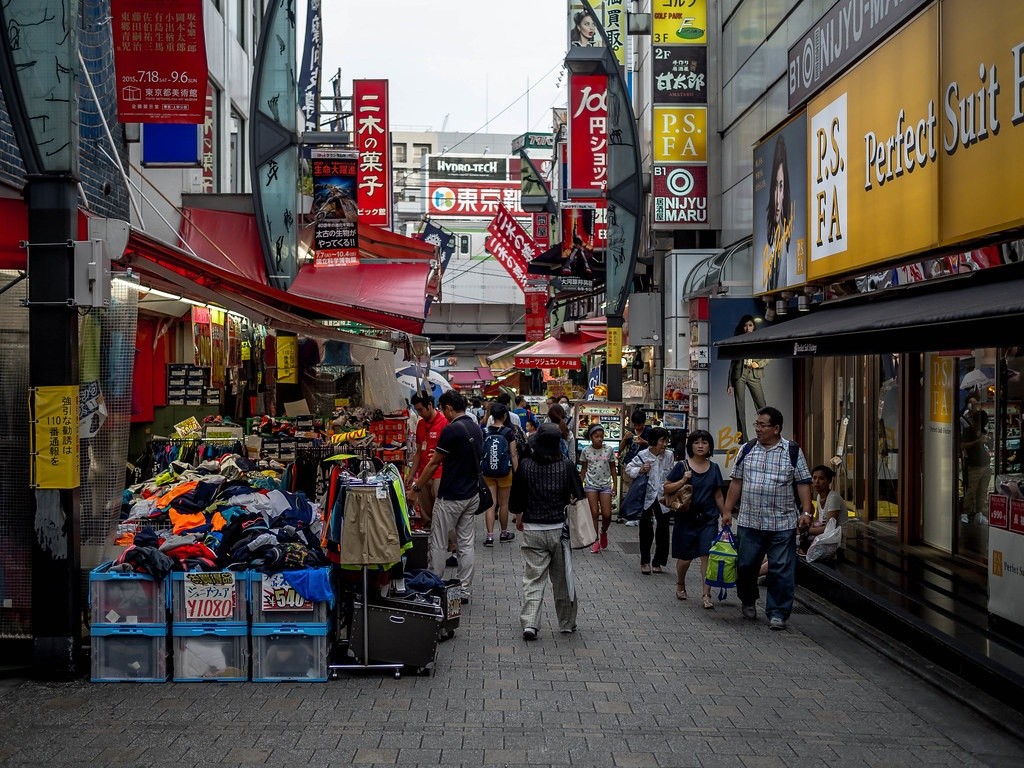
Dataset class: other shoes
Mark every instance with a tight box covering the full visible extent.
[653,566,662,573]
[560,624,577,633]
[523,628,538,640]
[961,513,969,523]
[641,563,651,574]
[974,512,988,526]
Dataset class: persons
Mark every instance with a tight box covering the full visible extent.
[465,392,539,437]
[625,427,675,574]
[405,390,458,567]
[664,430,724,607]
[508,423,587,638]
[724,406,812,629]
[571,11,595,47]
[544,395,576,464]
[579,423,617,554]
[957,410,991,527]
[759,465,848,577]
[765,135,791,291]
[407,390,483,604]
[618,410,652,527]
[726,314,772,444]
[481,403,527,547]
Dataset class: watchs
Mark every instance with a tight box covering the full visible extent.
[413,485,421,492]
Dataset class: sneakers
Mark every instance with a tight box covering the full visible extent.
[769,617,785,629]
[741,601,757,619]
[590,540,600,553]
[600,529,608,548]
[444,514,519,604]
[625,520,639,527]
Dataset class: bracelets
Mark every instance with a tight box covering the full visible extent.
[805,512,812,518]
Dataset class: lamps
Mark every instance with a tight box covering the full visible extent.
[775,291,794,315]
[555,83,567,88]
[589,41,596,47]
[549,276,563,291]
[561,64,566,70]
[762,295,776,322]
[572,40,583,47]
[631,345,645,370]
[797,285,817,312]
[483,147,489,156]
[441,145,447,156]
[558,77,563,82]
[560,70,564,76]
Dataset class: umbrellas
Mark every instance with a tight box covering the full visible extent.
[395,364,452,400]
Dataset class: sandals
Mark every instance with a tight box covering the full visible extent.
[703,595,713,609]
[674,580,688,599]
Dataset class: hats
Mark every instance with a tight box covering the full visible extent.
[528,422,563,455]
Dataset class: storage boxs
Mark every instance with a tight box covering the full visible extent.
[385,419,407,431]
[383,449,405,462]
[280,440,297,449]
[89,627,171,683]
[346,592,444,672]
[250,622,333,684]
[280,449,295,459]
[376,451,380,458]
[249,568,331,627]
[170,569,249,626]
[297,439,314,448]
[263,440,280,450]
[88,572,169,628]
[172,626,250,682]
[370,420,385,430]
[297,414,313,427]
[267,450,280,459]
[385,431,407,444]
[371,430,385,442]
[388,578,462,633]
[166,363,221,405]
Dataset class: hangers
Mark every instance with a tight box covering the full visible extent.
[294,445,378,464]
[145,437,241,447]
[338,470,390,492]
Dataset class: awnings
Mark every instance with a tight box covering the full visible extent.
[713,276,1024,360]
[77,205,442,354]
[514,336,606,370]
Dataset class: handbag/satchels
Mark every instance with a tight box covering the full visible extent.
[616,443,631,467]
[564,497,597,549]
[474,478,494,516]
[658,460,693,511]
[806,517,842,563]
[704,524,738,601]
[617,471,649,521]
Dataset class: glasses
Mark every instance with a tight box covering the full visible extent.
[753,422,775,429]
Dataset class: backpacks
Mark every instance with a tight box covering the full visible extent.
[480,426,512,478]
[472,408,485,421]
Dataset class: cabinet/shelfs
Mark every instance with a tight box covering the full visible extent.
[641,407,689,462]
[572,400,627,525]
